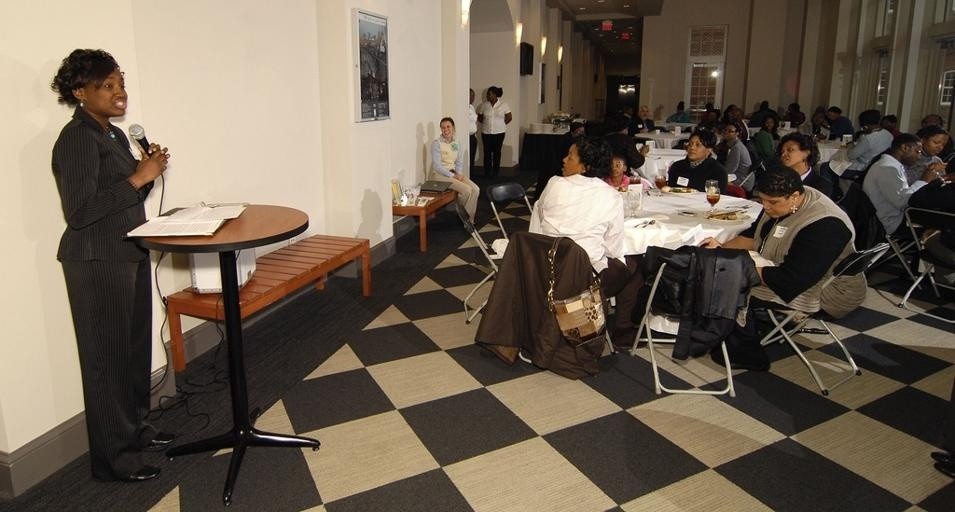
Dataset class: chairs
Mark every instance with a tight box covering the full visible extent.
[455,118,955,397]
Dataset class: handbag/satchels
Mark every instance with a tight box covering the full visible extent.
[547,237,607,348]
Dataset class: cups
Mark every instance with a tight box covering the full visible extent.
[629,176,641,184]
[628,184,643,201]
[705,180,719,192]
[656,129,660,137]
[842,135,853,146]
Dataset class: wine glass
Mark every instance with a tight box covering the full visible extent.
[655,174,667,198]
[626,192,640,219]
[706,186,720,213]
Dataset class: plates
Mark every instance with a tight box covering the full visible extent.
[669,187,700,198]
[529,123,554,134]
[703,209,748,222]
[623,218,662,232]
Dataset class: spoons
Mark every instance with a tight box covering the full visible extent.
[642,220,655,229]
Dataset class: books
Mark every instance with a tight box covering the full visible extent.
[126,206,247,237]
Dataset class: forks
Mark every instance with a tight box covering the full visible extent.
[634,219,648,228]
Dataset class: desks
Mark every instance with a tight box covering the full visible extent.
[136,205,321,507]
[393,190,458,252]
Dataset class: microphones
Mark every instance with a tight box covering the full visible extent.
[128,122,154,157]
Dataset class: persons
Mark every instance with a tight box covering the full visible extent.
[699,162,867,370]
[838,109,954,284]
[779,131,833,198]
[531,136,646,348]
[552,106,654,191]
[654,102,806,199]
[477,86,512,180]
[810,105,854,140]
[50,48,174,482]
[429,117,481,227]
[469,89,478,171]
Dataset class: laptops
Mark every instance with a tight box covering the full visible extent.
[421,181,453,193]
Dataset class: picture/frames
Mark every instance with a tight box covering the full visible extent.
[352,7,391,123]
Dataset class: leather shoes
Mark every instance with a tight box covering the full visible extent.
[930,452,951,462]
[143,432,176,452]
[130,465,161,481]
[934,461,954,478]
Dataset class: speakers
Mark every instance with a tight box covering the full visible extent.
[520,42,534,76]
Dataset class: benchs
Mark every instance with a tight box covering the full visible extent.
[167,235,371,373]
